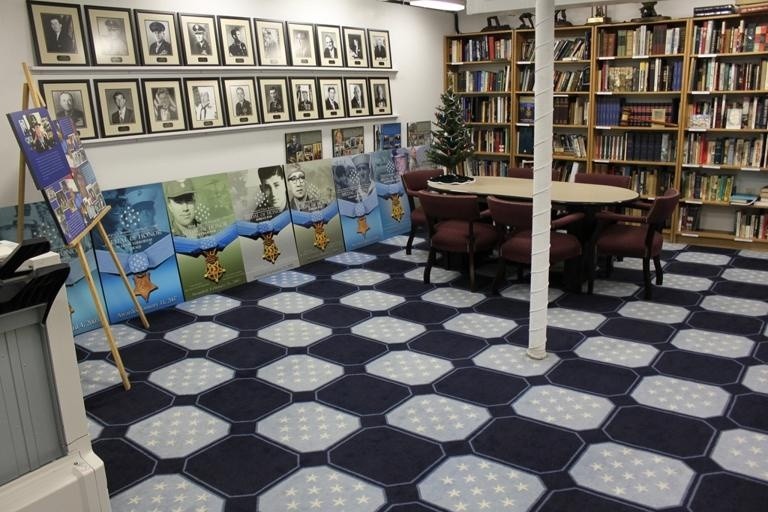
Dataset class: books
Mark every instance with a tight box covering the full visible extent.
[594,131,677,163]
[593,163,674,198]
[518,95,534,123]
[597,24,686,56]
[447,65,511,93]
[686,94,768,129]
[603,201,672,228]
[596,96,681,128]
[553,94,589,128]
[517,159,534,170]
[552,160,585,184]
[731,185,768,208]
[733,210,768,240]
[520,38,535,61]
[460,96,511,124]
[552,132,586,159]
[516,127,534,154]
[597,58,682,93]
[448,35,512,63]
[688,57,768,90]
[552,31,590,61]
[691,19,768,54]
[680,169,735,203]
[693,0,768,19]
[678,205,701,234]
[458,159,509,177]
[553,64,590,92]
[465,127,509,153]
[682,132,768,169]
[518,65,535,92]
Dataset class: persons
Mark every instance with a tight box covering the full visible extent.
[287,134,302,163]
[165,179,211,239]
[148,21,172,56]
[325,87,339,110]
[333,164,356,203]
[235,87,252,116]
[351,86,364,108]
[56,91,85,128]
[375,125,382,151]
[375,84,387,107]
[286,164,327,213]
[299,90,313,110]
[269,86,283,112]
[375,38,386,59]
[390,148,409,184]
[250,165,288,222]
[351,154,375,202]
[155,91,178,121]
[111,91,135,124]
[350,39,361,59]
[324,35,338,59]
[101,19,127,55]
[191,23,212,55]
[265,30,277,57]
[196,90,216,120]
[409,147,418,171]
[127,187,168,252]
[295,32,311,58]
[92,190,136,254]
[229,28,248,57]
[335,130,344,145]
[46,17,73,54]
[31,122,49,153]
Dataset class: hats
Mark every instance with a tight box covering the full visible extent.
[286,163,305,178]
[167,179,196,198]
[106,19,119,27]
[149,22,165,32]
[392,148,408,158]
[28,115,41,129]
[125,189,156,212]
[193,24,206,31]
[351,154,370,167]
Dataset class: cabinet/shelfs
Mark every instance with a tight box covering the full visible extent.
[673,11,768,251]
[596,20,687,242]
[28,65,397,143]
[442,29,513,175]
[515,27,593,180]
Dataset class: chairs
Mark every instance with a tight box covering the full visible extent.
[401,167,681,301]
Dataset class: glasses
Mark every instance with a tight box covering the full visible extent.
[105,198,126,207]
[288,175,305,181]
